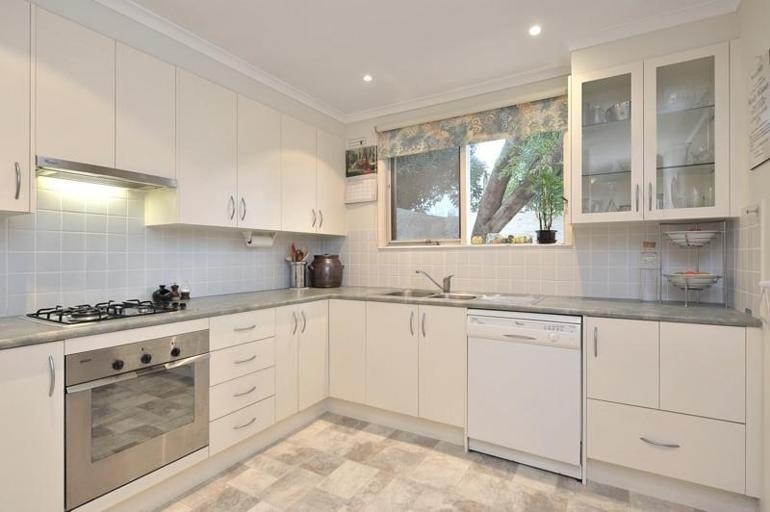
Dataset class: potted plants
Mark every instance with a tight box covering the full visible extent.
[522,162,568,244]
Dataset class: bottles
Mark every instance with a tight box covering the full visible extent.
[151,282,190,302]
[637,239,660,302]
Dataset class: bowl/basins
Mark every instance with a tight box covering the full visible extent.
[664,230,718,246]
[604,100,630,120]
[663,274,720,290]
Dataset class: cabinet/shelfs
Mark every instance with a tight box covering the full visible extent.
[27,0,182,190]
[284,77,349,243]
[364,299,471,456]
[273,296,332,442]
[0,1,37,223]
[0,335,69,512]
[140,30,284,237]
[208,302,275,476]
[328,296,366,423]
[564,13,745,228]
[581,394,768,511]
[581,311,768,424]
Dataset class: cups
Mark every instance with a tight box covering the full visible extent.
[585,104,600,124]
[291,262,308,289]
[584,192,664,212]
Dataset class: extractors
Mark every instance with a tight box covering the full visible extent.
[36,156,177,190]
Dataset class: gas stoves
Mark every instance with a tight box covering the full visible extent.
[28,298,180,327]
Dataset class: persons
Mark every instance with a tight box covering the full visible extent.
[350,150,372,170]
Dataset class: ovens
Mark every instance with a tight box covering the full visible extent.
[65,327,211,509]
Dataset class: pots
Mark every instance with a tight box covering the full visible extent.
[309,252,345,288]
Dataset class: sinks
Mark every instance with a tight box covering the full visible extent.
[383,288,439,306]
[430,294,477,306]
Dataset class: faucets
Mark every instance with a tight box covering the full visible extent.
[416,269,455,293]
